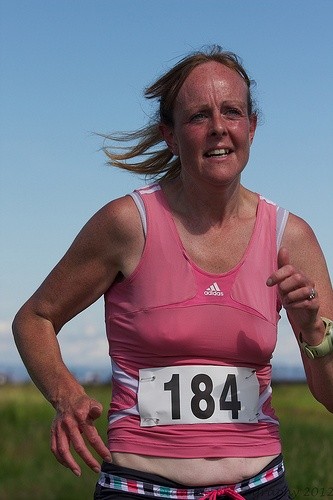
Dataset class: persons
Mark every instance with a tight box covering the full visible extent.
[12,45,333,500]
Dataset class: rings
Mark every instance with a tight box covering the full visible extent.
[309,287,315,299]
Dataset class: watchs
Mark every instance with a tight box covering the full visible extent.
[294,316,333,360]
